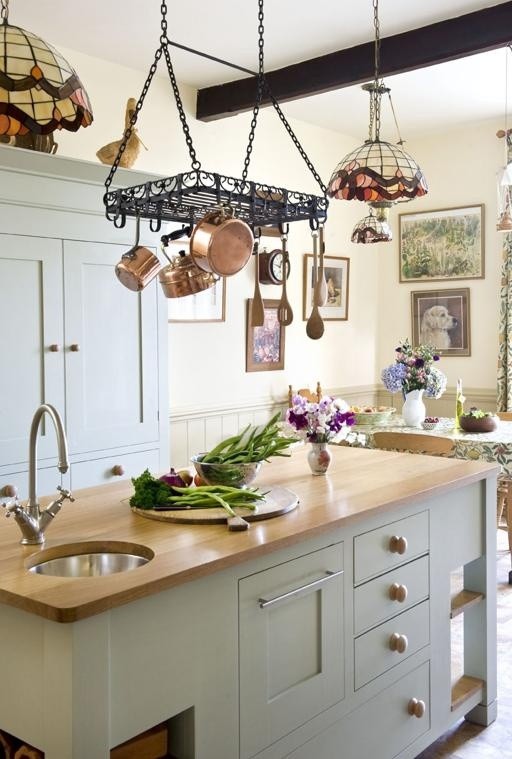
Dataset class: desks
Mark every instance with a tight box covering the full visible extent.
[344,415,512,475]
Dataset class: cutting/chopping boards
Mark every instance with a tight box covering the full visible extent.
[130,487,300,530]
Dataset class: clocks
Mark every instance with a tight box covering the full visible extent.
[259,249,290,285]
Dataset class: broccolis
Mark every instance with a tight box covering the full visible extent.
[130,467,172,510]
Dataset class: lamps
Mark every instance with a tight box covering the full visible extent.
[328,0,428,244]
[0,0,94,140]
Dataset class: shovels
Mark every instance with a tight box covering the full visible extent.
[314,227,328,306]
[251,242,264,327]
[306,234,324,339]
[276,239,293,327]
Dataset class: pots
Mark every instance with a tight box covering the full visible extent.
[113,206,254,292]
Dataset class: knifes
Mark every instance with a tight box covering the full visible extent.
[150,504,207,512]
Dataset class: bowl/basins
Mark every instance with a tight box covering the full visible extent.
[353,407,396,425]
[189,453,264,488]
[459,414,501,432]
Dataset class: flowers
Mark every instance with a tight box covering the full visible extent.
[381,339,447,400]
[286,395,366,446]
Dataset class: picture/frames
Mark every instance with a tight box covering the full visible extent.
[303,254,350,320]
[246,297,284,371]
[411,288,470,355]
[398,202,485,283]
[168,240,225,322]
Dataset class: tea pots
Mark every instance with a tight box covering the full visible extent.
[158,227,213,299]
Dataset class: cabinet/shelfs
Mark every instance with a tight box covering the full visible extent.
[0,144,171,464]
[238,479,484,759]
[0,449,170,504]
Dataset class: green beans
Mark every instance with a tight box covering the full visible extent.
[168,485,271,517]
[202,411,300,474]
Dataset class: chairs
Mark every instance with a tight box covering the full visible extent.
[373,432,455,456]
[289,381,322,407]
[495,412,512,550]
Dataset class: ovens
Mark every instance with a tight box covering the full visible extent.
[236,540,346,758]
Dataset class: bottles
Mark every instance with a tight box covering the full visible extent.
[455,378,463,429]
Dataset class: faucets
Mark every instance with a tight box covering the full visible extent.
[26,404,68,517]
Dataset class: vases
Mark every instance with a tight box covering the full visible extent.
[402,388,425,427]
[308,443,330,475]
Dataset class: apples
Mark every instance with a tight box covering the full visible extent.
[194,474,206,486]
[178,470,193,486]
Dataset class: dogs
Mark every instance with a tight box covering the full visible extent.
[420,306,457,348]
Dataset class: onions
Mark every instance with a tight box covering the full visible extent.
[159,467,186,496]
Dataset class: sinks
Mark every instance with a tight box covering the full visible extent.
[25,541,157,577]
